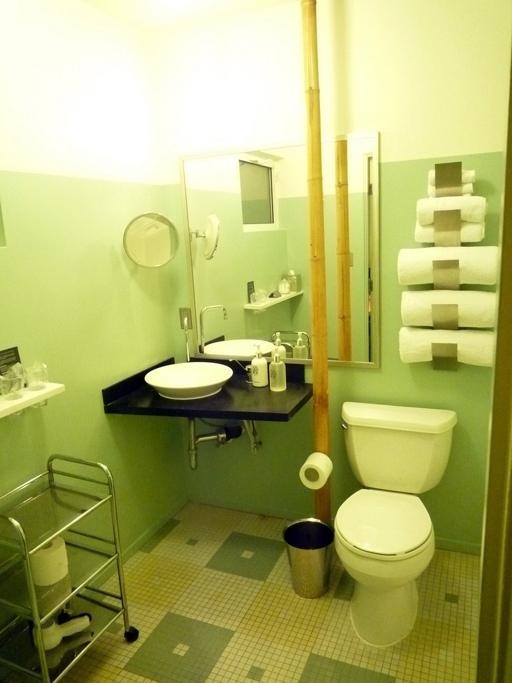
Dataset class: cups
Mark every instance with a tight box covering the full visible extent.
[0,364,51,400]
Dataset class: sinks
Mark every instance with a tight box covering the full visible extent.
[204,338,274,357]
[144,361,234,401]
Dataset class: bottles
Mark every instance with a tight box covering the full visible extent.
[278,268,298,294]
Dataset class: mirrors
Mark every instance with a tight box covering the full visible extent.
[182,130,381,372]
[122,211,179,269]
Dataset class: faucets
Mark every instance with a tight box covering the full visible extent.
[229,358,252,384]
[183,316,191,362]
[199,305,228,354]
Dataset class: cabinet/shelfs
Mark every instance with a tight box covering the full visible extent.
[0,453,140,681]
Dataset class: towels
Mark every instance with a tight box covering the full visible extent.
[395,170,500,367]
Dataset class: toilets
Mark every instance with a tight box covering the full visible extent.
[333,401,458,646]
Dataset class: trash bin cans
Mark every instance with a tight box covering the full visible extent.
[283,517,334,599]
